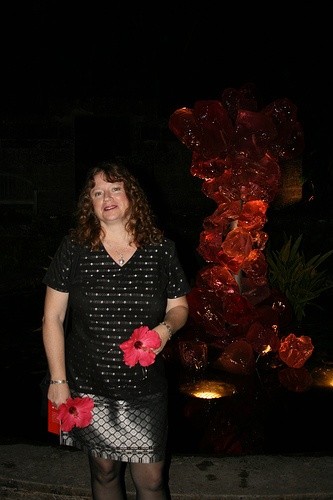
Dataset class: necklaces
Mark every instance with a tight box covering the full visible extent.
[100,219,134,266]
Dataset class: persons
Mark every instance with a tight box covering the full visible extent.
[41,156,192,500]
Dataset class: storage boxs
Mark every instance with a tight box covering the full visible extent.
[48,398,61,435]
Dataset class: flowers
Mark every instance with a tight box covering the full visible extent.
[59,397,94,431]
[119,325,161,367]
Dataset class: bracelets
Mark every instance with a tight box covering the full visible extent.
[50,379,68,384]
[162,322,176,336]
[160,323,172,340]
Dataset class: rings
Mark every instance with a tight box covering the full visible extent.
[51,401,59,410]
